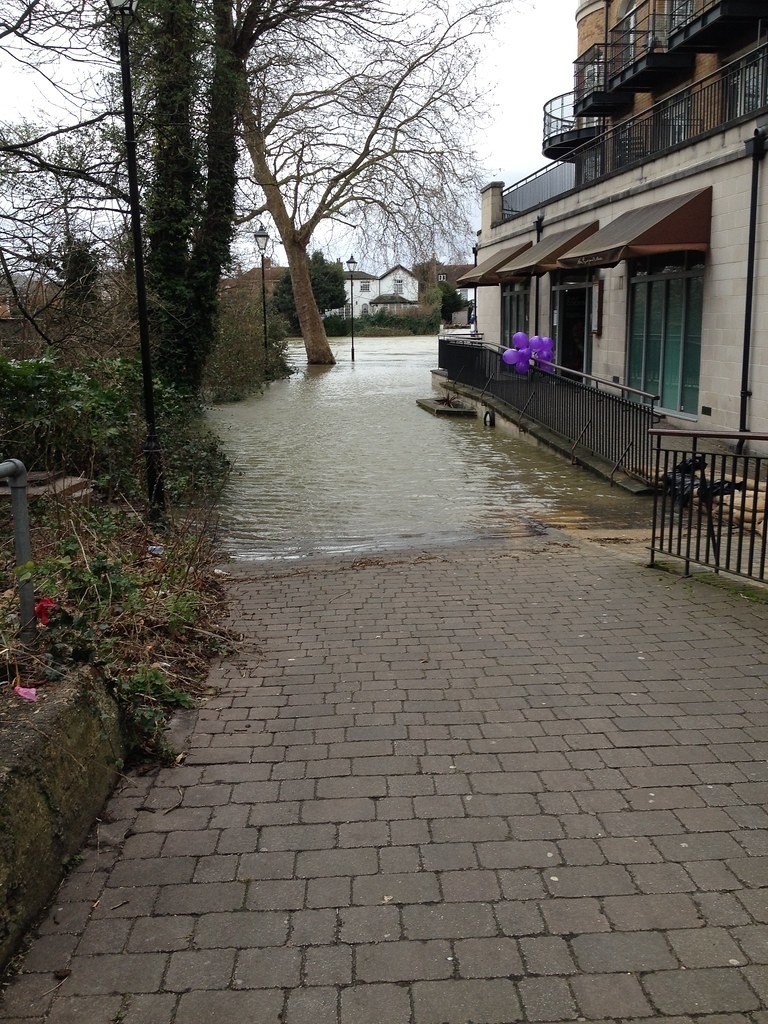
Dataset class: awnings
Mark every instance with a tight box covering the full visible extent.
[457,220,599,285]
[557,186,712,267]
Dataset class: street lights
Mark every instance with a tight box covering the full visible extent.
[254,221,273,380]
[345,253,361,362]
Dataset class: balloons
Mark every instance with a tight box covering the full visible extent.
[502,332,553,374]
